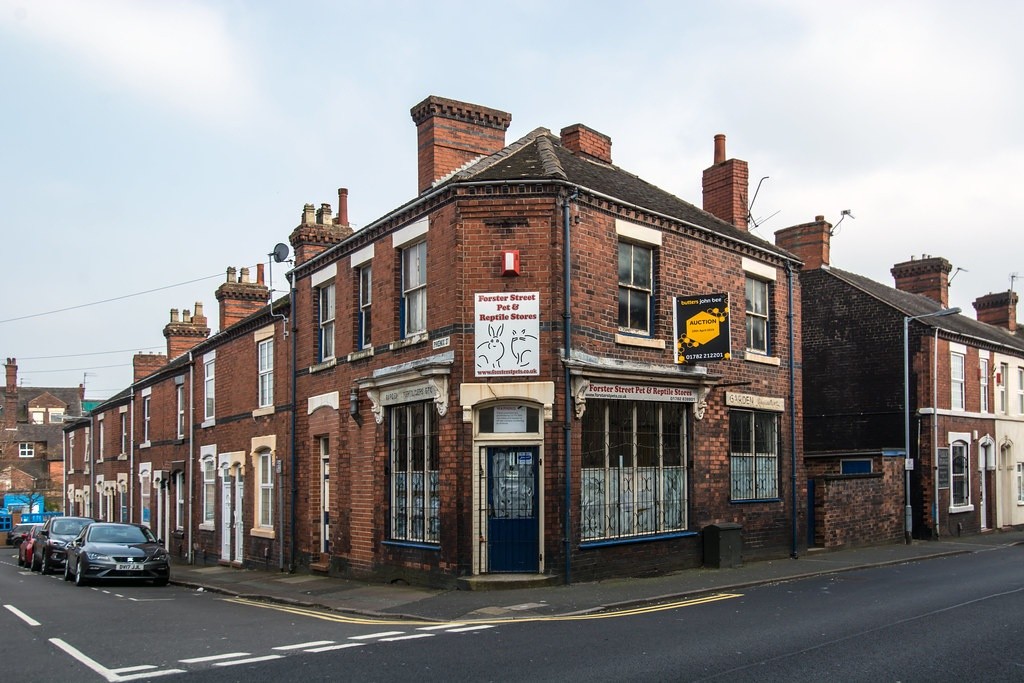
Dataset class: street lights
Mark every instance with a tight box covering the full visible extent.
[902,305,964,544]
[57,415,95,518]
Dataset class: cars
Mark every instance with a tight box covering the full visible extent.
[30,516,104,575]
[18,523,49,568]
[6,523,38,548]
[64,522,171,587]
